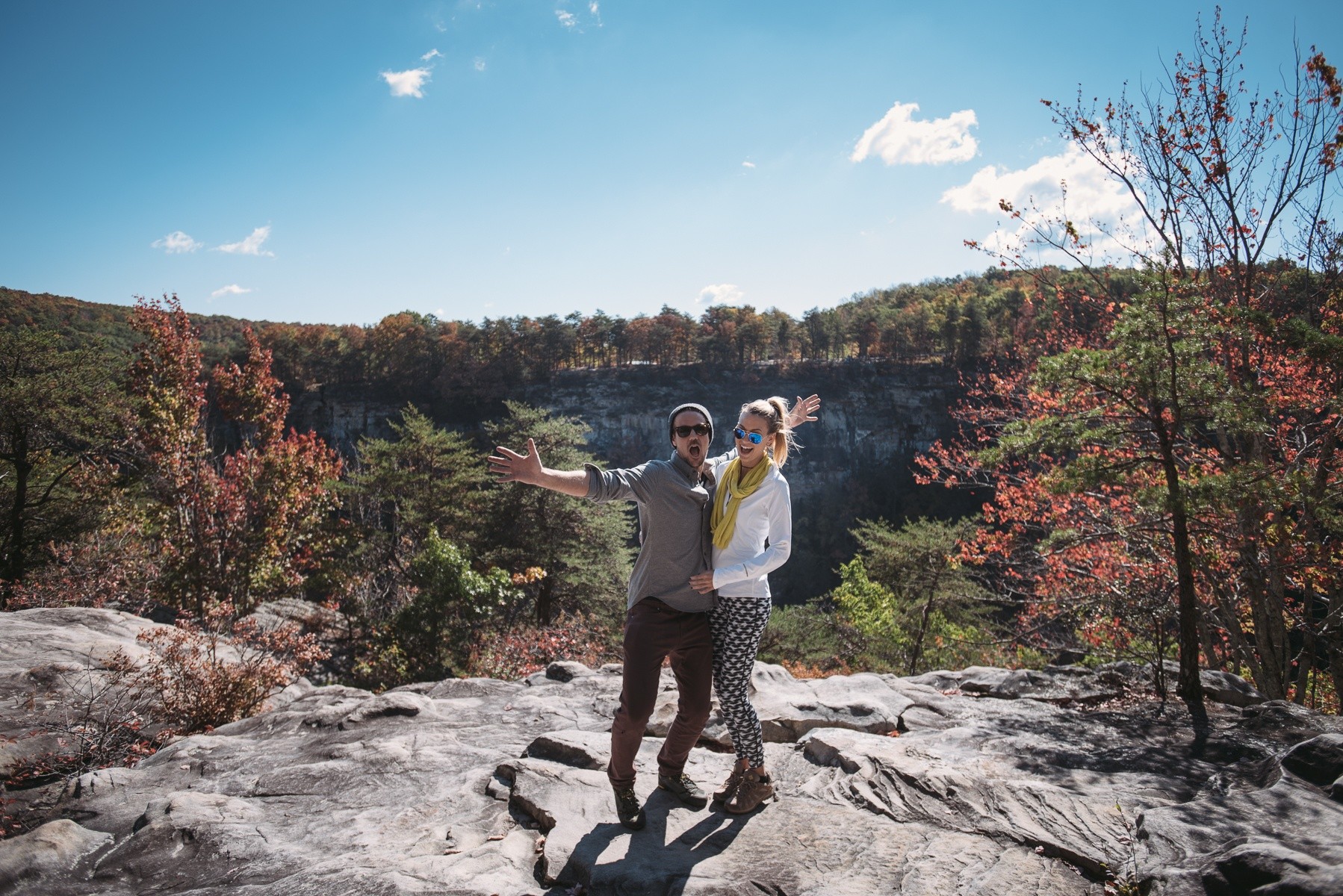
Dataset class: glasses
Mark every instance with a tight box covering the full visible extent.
[733,427,770,444]
[674,423,710,437]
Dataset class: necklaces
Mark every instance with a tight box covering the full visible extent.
[741,465,752,469]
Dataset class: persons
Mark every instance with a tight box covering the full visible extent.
[688,397,792,814]
[488,394,823,832]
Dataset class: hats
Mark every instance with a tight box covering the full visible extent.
[669,403,714,449]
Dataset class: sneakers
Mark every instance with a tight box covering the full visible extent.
[713,769,745,801]
[659,772,708,807]
[725,769,775,814]
[614,788,646,830]
